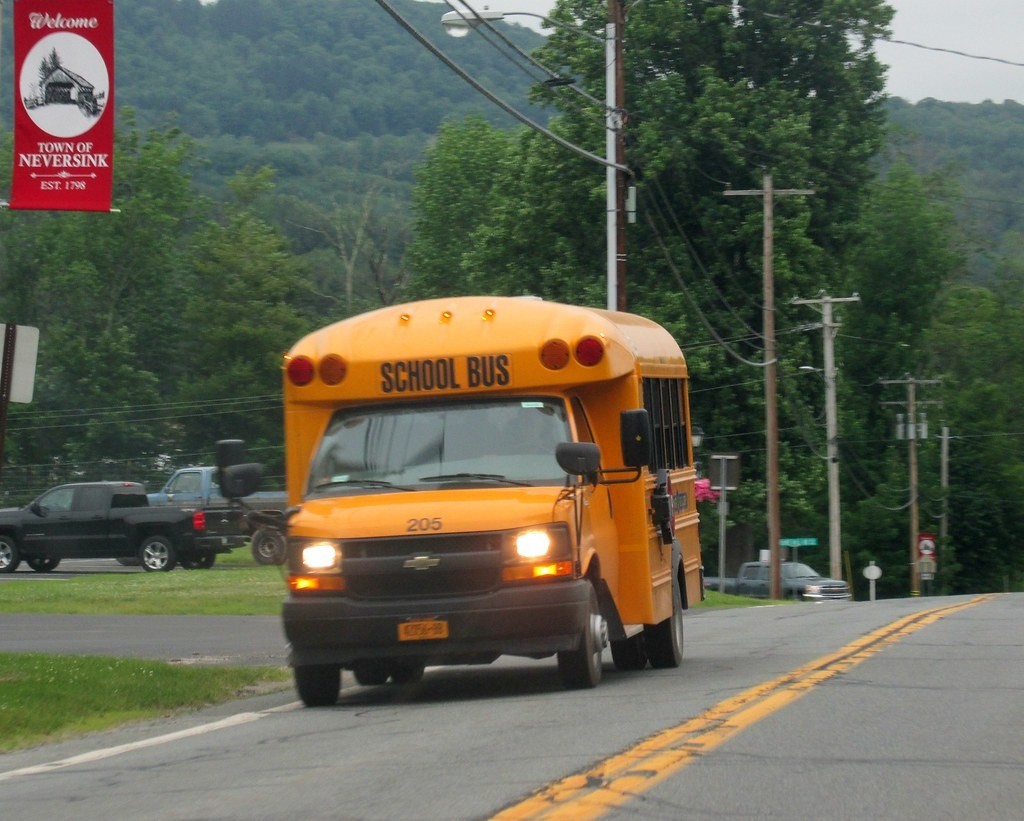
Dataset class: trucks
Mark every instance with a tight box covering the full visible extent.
[146,466,288,565]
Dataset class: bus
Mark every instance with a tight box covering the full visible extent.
[211,295,704,708]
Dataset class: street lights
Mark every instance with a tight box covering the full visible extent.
[690,421,705,554]
[799,366,842,580]
[441,10,617,313]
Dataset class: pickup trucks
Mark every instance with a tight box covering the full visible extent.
[703,561,852,602]
[0,482,252,573]
